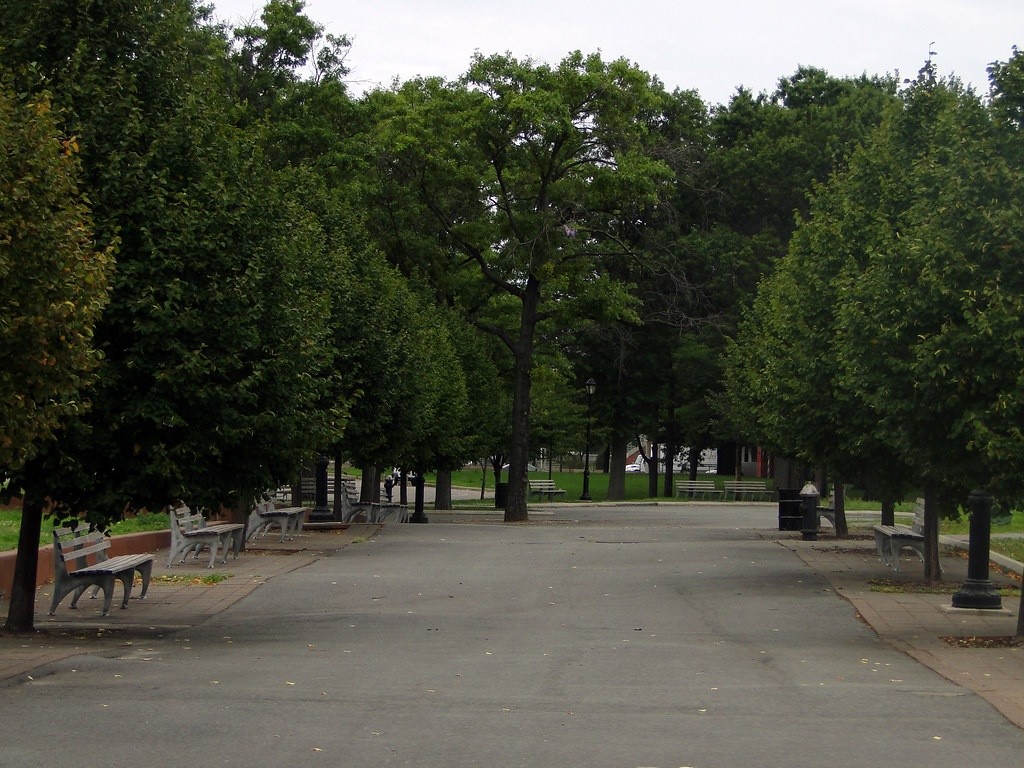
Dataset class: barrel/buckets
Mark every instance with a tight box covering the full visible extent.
[776,486,803,532]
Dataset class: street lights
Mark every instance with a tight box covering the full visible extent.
[579,377,597,499]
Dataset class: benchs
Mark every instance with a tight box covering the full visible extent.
[675,480,724,501]
[246,488,307,543]
[276,477,359,507]
[527,480,568,503]
[723,480,775,502]
[341,482,383,524]
[46,523,155,617]
[377,487,409,523]
[817,482,849,530]
[873,497,945,574]
[166,500,245,569]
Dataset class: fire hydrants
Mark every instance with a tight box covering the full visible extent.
[800,484,820,539]
